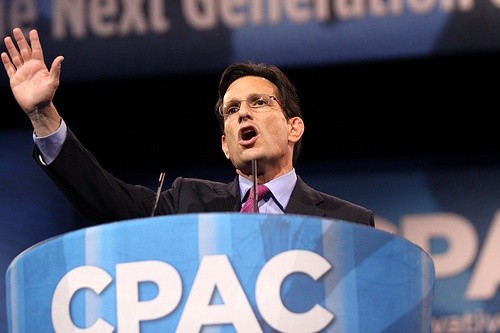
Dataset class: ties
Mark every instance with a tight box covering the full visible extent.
[240,185,270,214]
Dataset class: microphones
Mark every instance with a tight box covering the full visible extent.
[251,160,258,214]
[152,173,165,216]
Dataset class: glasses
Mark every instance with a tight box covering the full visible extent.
[216,94,291,118]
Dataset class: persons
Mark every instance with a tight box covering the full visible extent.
[1,27,376,228]
